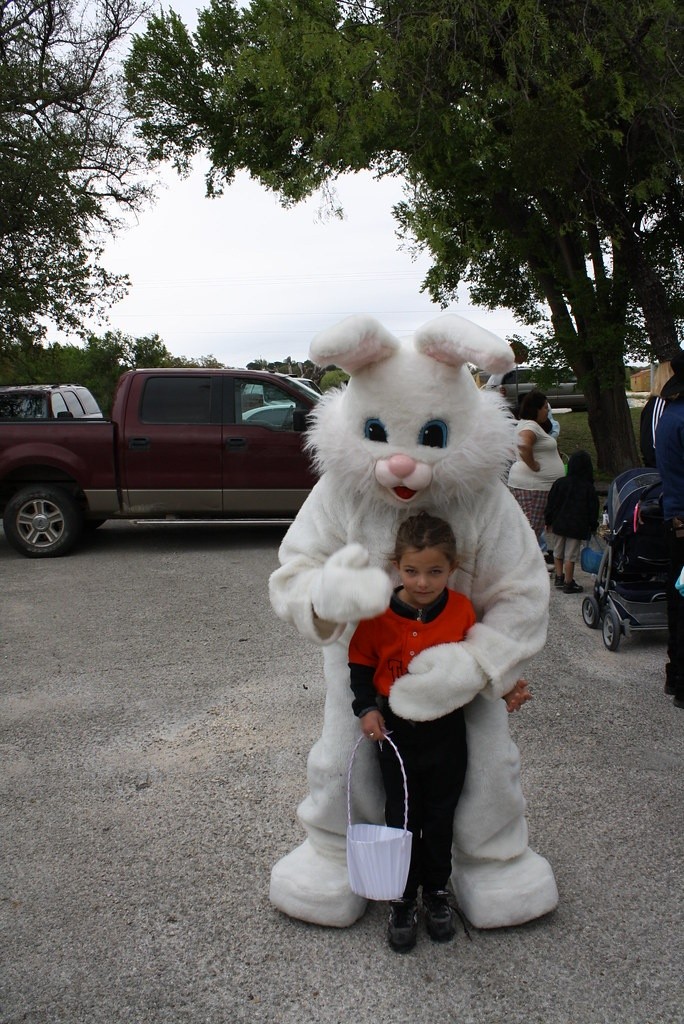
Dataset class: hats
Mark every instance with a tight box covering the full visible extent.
[660,351,684,399]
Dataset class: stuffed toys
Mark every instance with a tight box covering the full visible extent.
[268,314,559,930]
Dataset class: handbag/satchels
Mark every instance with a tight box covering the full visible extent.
[346,733,413,902]
[581,534,604,575]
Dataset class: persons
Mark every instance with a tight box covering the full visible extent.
[654,350,684,709]
[347,514,533,955]
[545,450,600,594]
[508,389,565,582]
[640,361,674,468]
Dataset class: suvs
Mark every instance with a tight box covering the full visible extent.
[1,384,103,422]
[242,369,324,432]
[486,366,588,412]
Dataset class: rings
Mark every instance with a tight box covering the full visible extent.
[369,733,375,737]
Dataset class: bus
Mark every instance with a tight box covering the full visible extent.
[472,371,490,387]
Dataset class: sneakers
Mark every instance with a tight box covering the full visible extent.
[421,889,473,942]
[387,902,418,953]
[563,579,583,594]
[554,574,565,586]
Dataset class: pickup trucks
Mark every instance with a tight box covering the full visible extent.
[1,367,329,558]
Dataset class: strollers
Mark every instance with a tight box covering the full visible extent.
[580,470,667,649]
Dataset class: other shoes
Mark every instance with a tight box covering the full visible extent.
[673,693,684,709]
[664,680,684,695]
